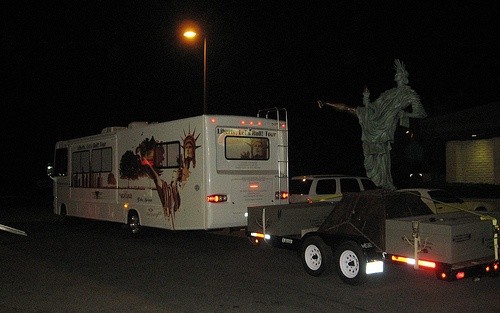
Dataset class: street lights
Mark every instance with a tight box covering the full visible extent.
[183,29,207,114]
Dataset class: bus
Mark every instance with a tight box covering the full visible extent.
[47,108,290,242]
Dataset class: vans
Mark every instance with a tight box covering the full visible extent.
[290,174,384,203]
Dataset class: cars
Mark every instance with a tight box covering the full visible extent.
[394,188,496,215]
